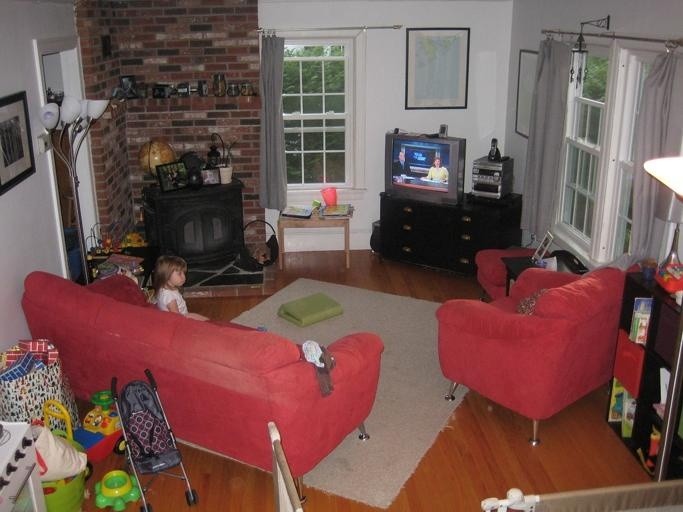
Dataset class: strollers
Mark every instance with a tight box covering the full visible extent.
[103,366,205,512]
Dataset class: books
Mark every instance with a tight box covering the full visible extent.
[608,298,683,476]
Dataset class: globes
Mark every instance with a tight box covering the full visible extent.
[138,139,176,187]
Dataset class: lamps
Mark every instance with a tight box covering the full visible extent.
[640,144,681,489]
[31,85,108,282]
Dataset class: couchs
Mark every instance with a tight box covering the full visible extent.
[433,262,637,445]
[476,245,554,300]
[23,264,383,507]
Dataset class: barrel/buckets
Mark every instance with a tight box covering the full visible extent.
[321,188,338,207]
[215,164,234,186]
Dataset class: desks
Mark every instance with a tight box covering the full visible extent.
[499,249,587,296]
[0,420,49,512]
[276,206,352,269]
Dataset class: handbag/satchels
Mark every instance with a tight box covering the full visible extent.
[0,361,87,483]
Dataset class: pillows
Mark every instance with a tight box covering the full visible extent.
[516,286,552,315]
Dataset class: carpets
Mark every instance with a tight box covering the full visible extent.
[172,274,471,510]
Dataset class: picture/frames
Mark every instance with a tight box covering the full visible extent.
[118,74,141,99]
[403,26,471,110]
[532,230,554,263]
[0,90,37,199]
[200,165,220,187]
[153,160,190,192]
[511,46,543,139]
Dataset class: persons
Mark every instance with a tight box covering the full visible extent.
[154,254,207,320]
[423,158,449,184]
[392,150,411,177]
[172,170,180,189]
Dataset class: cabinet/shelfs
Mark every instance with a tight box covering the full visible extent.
[377,191,522,278]
[605,269,682,484]
[141,176,245,272]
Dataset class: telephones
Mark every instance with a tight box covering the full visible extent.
[488,138,501,161]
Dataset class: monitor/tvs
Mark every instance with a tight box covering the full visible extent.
[385,132,466,205]
